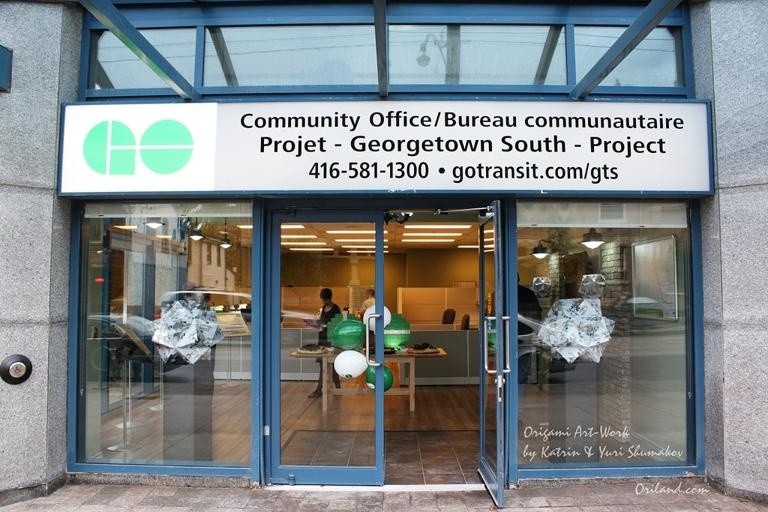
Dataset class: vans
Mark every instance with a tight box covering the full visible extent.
[161,290,284,341]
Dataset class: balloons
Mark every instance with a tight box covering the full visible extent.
[332,304,411,393]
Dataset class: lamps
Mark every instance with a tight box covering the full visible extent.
[529,239,553,260]
[580,228,606,249]
[188,217,233,249]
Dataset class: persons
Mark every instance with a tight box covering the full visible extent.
[307,287,342,399]
[489,271,542,429]
[359,289,376,345]
[186,284,217,409]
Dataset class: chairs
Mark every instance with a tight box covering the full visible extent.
[442,308,470,330]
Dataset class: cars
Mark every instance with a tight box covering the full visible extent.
[484,314,581,384]
[86,315,193,384]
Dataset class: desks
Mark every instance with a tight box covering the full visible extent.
[289,344,449,412]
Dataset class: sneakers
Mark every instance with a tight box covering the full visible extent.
[307,390,322,398]
